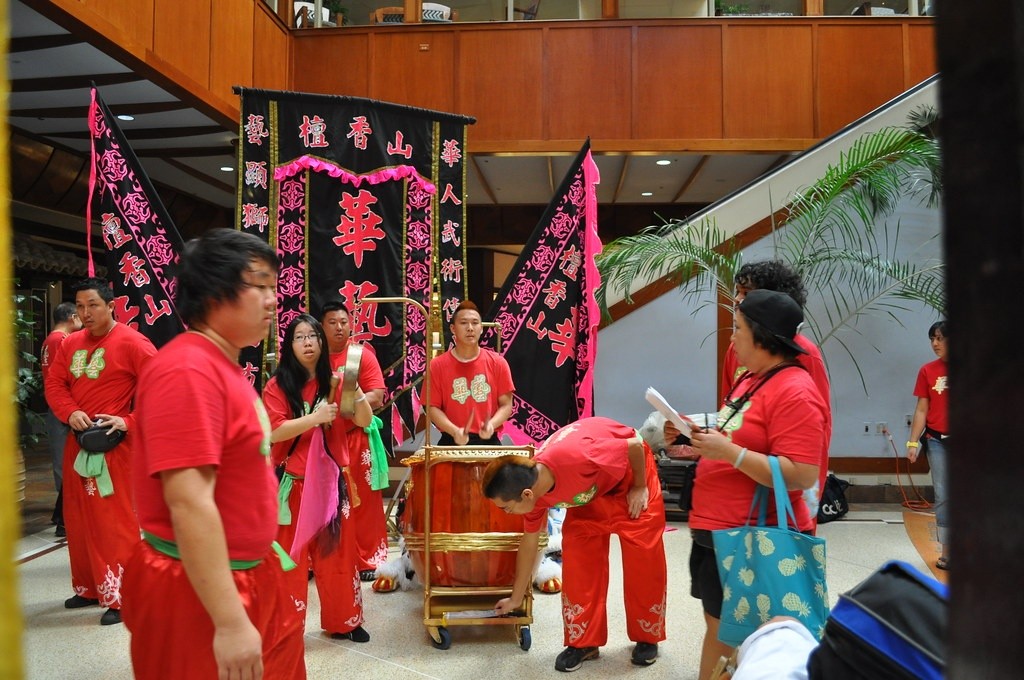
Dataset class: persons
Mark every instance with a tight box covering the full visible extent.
[722,262,832,441]
[372,302,562,594]
[307,304,385,580]
[906,321,950,569]
[45,278,158,625]
[664,290,829,680]
[40,303,82,536]
[263,315,372,641]
[120,231,308,680]
[483,417,668,671]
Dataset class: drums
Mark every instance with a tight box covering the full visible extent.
[402,444,549,587]
[339,342,364,419]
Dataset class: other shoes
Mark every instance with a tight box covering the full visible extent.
[51,518,59,524]
[53,522,65,537]
[936,558,951,570]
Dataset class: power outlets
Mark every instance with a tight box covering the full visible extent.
[904,413,912,427]
[874,422,888,434]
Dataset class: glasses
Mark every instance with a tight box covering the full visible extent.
[291,333,321,342]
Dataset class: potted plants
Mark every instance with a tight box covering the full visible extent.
[6,276,47,515]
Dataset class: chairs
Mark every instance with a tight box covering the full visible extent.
[294,3,458,29]
[490,0,540,21]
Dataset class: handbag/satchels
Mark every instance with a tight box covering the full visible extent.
[72,421,127,452]
[710,456,830,648]
[817,473,849,524]
[806,560,948,680]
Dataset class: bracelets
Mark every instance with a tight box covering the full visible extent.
[355,385,360,391]
[734,448,747,469]
[907,441,918,448]
[354,393,366,403]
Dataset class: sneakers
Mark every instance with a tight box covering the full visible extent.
[65,594,99,609]
[331,624,370,642]
[101,608,121,625]
[630,642,658,666]
[555,645,599,672]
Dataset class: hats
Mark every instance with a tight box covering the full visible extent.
[738,289,810,356]
